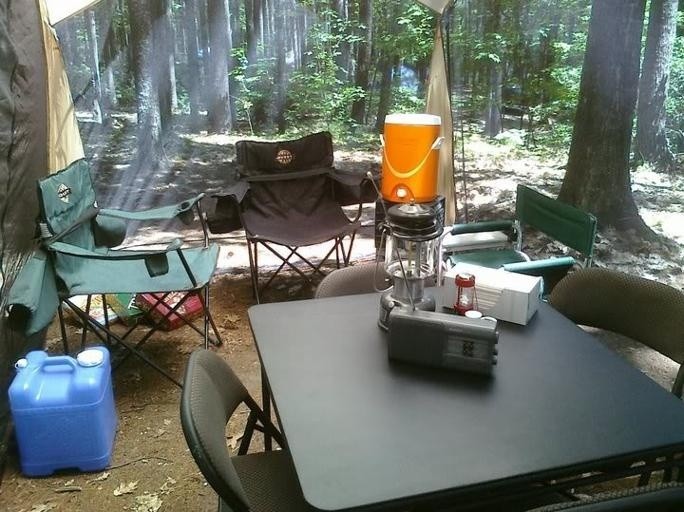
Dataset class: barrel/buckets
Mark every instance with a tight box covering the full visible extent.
[8,345,117,477]
[379,114,445,203]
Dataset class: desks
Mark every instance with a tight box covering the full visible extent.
[247,286,683,512]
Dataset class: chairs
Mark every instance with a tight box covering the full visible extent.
[35,156,224,388]
[532,482,684,512]
[440,185,597,304]
[180,347,322,512]
[315,261,438,299]
[549,267,683,486]
[207,131,377,303]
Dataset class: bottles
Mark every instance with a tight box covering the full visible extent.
[454,273,475,316]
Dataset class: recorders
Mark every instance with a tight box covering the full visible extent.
[386,303,500,380]
